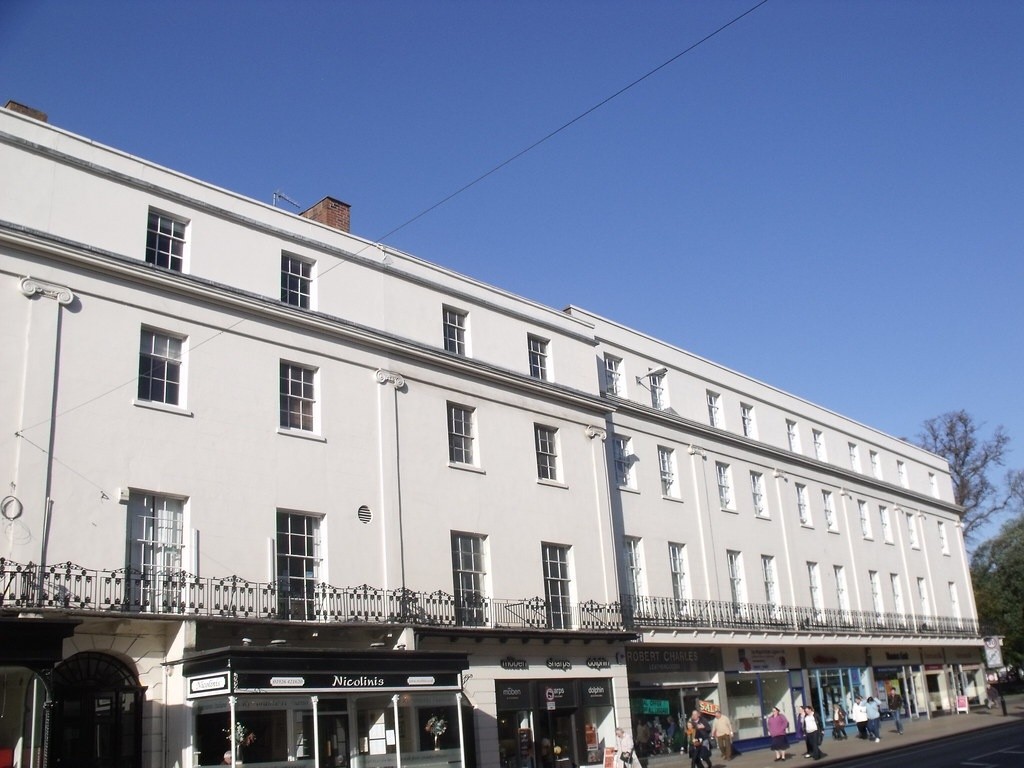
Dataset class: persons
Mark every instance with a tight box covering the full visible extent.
[767,707,789,761]
[686,722,704,768]
[668,716,674,737]
[888,687,903,734]
[833,703,847,740]
[804,706,819,761]
[852,695,882,743]
[691,710,711,768]
[796,705,807,754]
[712,711,734,761]
[811,706,824,735]
[637,718,648,743]
[985,683,998,708]
[221,751,232,765]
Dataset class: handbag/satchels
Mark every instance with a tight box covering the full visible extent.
[839,721,845,726]
[620,752,633,763]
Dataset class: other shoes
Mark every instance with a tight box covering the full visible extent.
[805,754,812,758]
[875,738,881,743]
[774,756,781,762]
[781,756,785,761]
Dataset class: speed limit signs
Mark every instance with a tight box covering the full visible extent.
[986,638,997,648]
